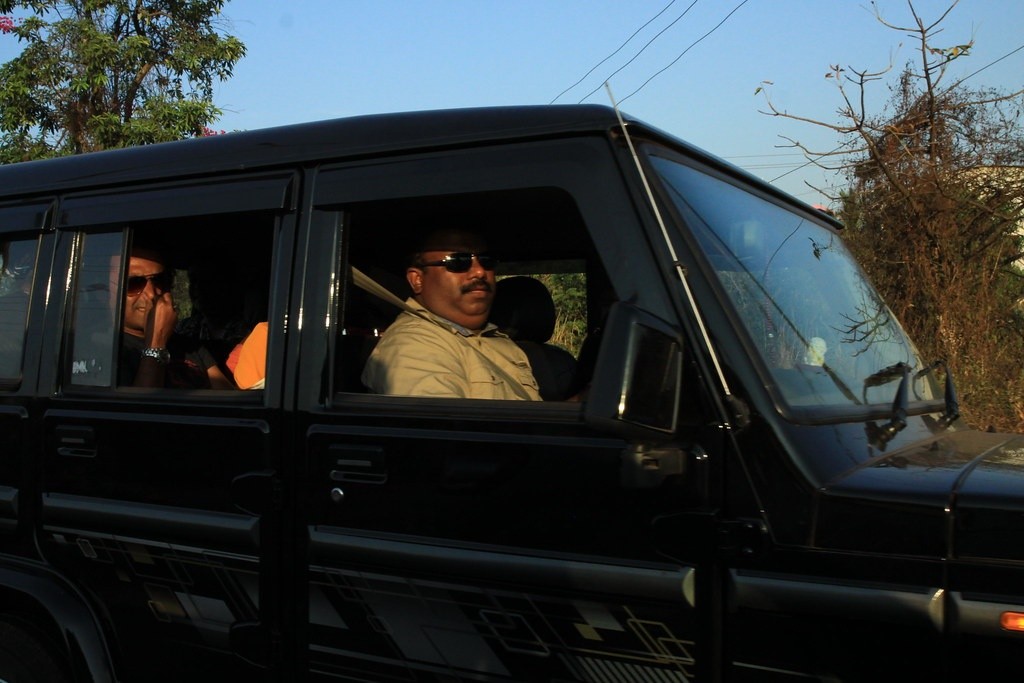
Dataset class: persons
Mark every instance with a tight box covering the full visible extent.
[107,241,208,389]
[361,207,578,407]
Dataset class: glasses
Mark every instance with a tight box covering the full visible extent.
[419,253,498,273]
[126,272,174,294]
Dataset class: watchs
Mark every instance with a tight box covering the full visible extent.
[143,347,172,364]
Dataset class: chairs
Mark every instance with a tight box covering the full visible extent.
[488,276,579,403]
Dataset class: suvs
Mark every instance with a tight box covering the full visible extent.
[0,104,1024,683]
